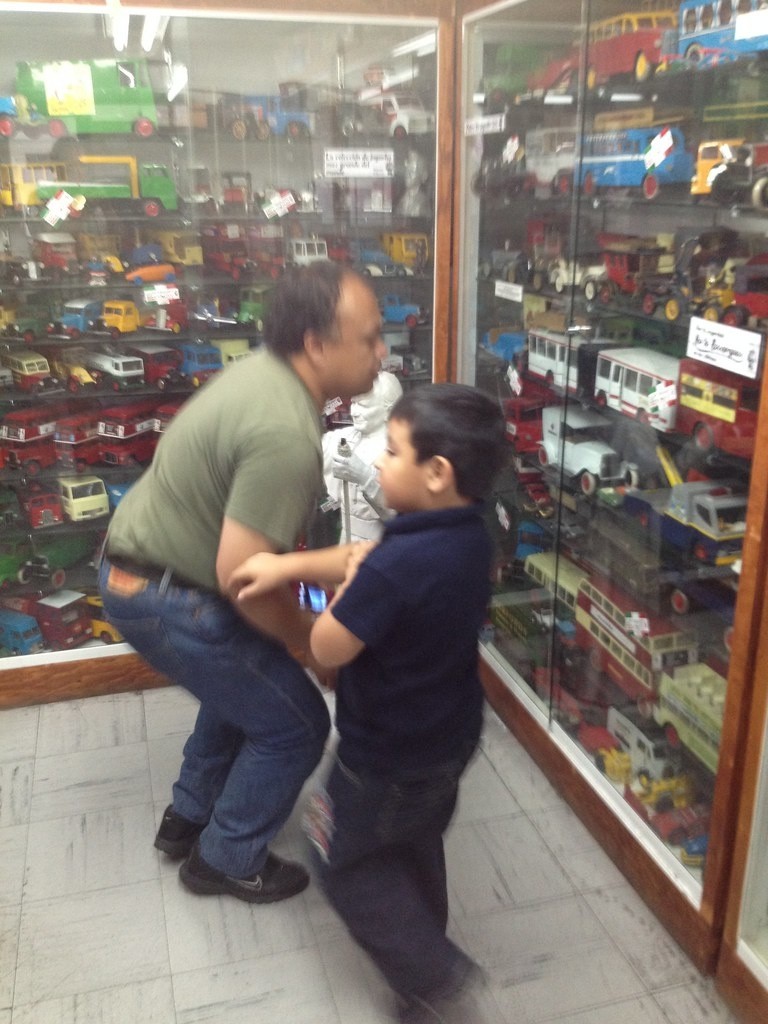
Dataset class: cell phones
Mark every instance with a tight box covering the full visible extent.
[296,582,328,613]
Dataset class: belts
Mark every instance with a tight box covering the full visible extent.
[104,545,201,589]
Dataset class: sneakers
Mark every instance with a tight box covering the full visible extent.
[179,838,309,904]
[155,805,207,855]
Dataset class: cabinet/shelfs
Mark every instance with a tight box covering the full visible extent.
[0,0,768,976]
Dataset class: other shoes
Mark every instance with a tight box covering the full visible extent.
[396,937,487,1024]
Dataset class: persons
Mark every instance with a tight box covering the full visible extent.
[229,383,509,1024]
[95,262,387,900]
[317,372,404,552]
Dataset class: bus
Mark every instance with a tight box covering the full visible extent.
[478,1,768,868]
[0,53,435,655]
